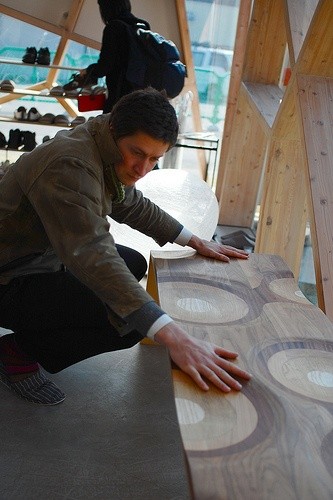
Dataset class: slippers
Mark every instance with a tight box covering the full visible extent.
[0,333,67,405]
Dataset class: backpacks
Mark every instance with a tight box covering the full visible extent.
[107,17,187,99]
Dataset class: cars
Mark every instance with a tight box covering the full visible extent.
[191,43,233,102]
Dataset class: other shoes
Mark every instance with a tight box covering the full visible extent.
[50,74,104,97]
[39,113,55,123]
[21,131,37,152]
[54,115,69,126]
[0,132,6,147]
[28,108,42,121]
[0,79,14,93]
[8,129,21,150]
[22,47,39,64]
[71,116,85,127]
[14,106,28,121]
[38,48,50,65]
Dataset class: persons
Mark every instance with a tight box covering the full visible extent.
[79,0,160,170]
[0,87,253,406]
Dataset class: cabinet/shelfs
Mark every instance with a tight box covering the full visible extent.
[0,58,84,180]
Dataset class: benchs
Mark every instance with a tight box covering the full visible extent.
[140,250,333,500]
